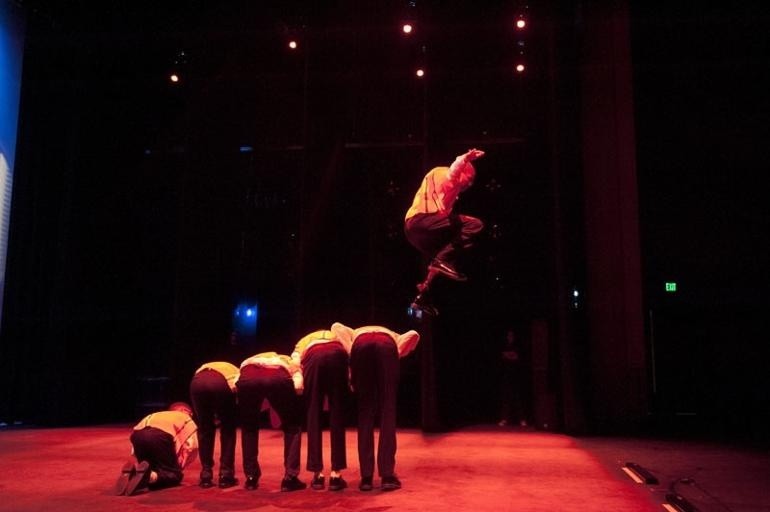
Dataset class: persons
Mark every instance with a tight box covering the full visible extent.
[402,146,488,321]
[236,350,306,492]
[497,327,529,427]
[114,400,200,496]
[288,326,352,490]
[331,319,421,491]
[189,360,242,489]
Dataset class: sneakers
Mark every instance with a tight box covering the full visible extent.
[411,296,438,316]
[427,258,468,282]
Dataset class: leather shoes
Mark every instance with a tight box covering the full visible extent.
[520,420,528,428]
[114,462,136,496]
[127,461,151,496]
[497,418,512,427]
[200,473,403,492]
